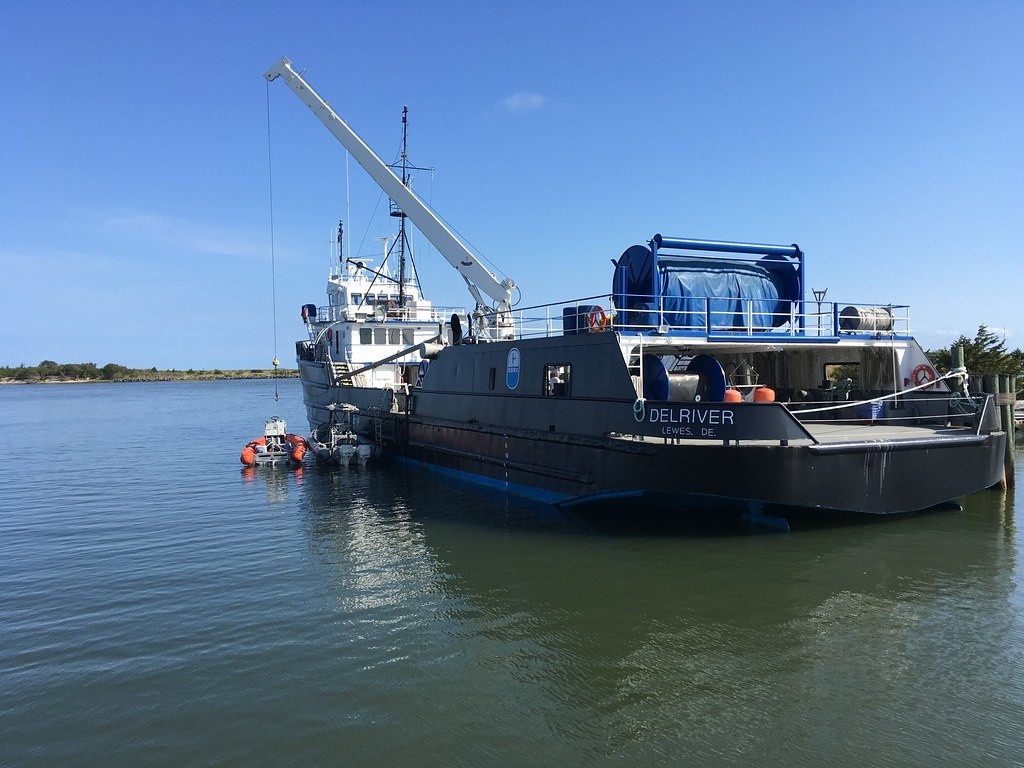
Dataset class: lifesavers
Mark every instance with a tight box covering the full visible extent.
[913,365,936,389]
[587,306,605,333]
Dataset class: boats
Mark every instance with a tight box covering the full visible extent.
[306,421,377,466]
[295,105,1005,517]
[241,416,307,465]
